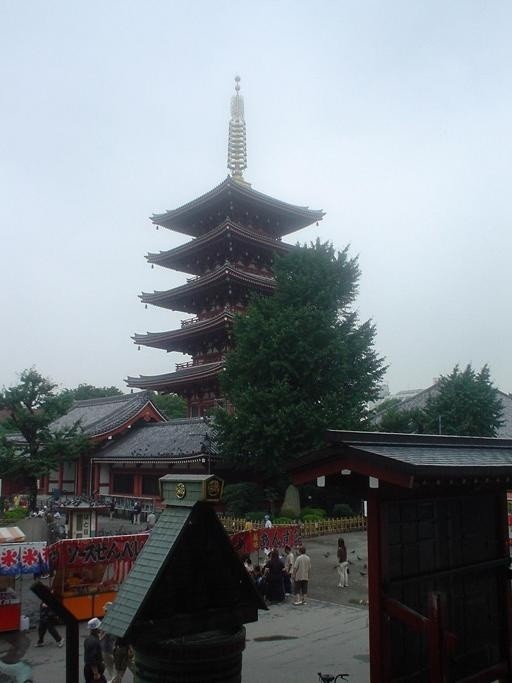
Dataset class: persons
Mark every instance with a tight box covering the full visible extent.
[264,514,273,527]
[132,502,140,524]
[83,617,107,682]
[337,537,349,587]
[244,516,253,530]
[13,494,20,507]
[110,639,136,682]
[34,588,64,647]
[244,543,312,605]
[99,601,114,677]
[109,498,115,519]
[146,510,156,529]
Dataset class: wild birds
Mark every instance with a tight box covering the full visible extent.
[322,550,367,576]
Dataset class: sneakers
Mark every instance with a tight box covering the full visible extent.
[35,643,44,647]
[58,638,65,648]
[292,598,308,605]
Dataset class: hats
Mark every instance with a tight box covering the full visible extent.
[87,618,102,630]
[103,602,112,610]
